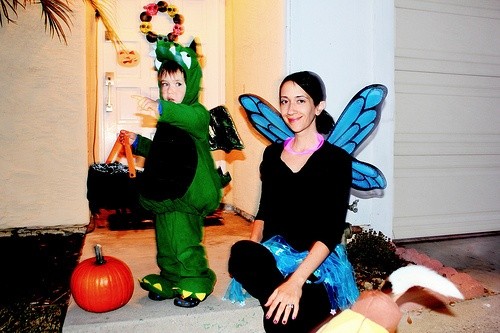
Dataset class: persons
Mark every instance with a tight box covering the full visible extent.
[118,38,223,308]
[228,71,359,333]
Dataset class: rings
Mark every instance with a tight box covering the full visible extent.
[286,304,293,308]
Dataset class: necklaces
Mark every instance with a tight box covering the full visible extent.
[284,133,324,155]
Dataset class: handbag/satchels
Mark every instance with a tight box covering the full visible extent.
[87,134,146,210]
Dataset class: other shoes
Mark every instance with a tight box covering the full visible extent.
[173,296,199,308]
[147,291,162,301]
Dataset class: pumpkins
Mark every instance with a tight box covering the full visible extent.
[70,244,134,313]
